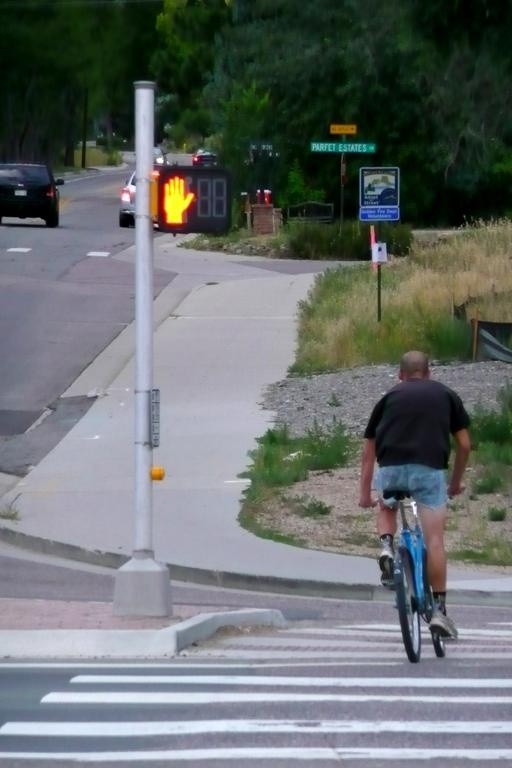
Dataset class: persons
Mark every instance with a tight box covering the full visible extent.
[359,351,473,639]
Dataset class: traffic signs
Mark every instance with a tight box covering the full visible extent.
[310,142,376,154]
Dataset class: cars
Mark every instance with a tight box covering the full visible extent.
[153,146,170,169]
[192,145,219,167]
[0,163,65,228]
[119,168,136,228]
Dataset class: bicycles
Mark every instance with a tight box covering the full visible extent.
[359,483,465,663]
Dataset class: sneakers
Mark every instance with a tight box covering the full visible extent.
[429,609,459,640]
[378,545,395,590]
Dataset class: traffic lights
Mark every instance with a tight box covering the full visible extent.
[151,166,232,234]
